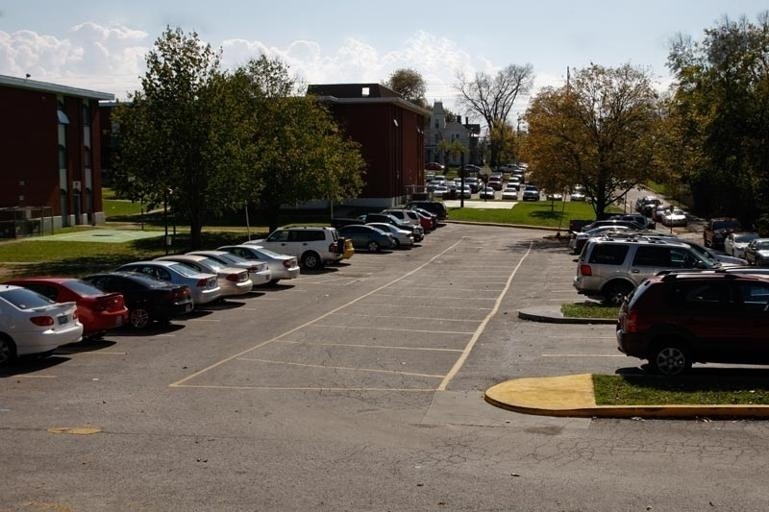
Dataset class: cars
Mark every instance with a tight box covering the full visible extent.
[570,195,768,266]
[422,159,587,201]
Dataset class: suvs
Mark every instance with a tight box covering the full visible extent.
[573,236,749,304]
[616,270,769,377]
